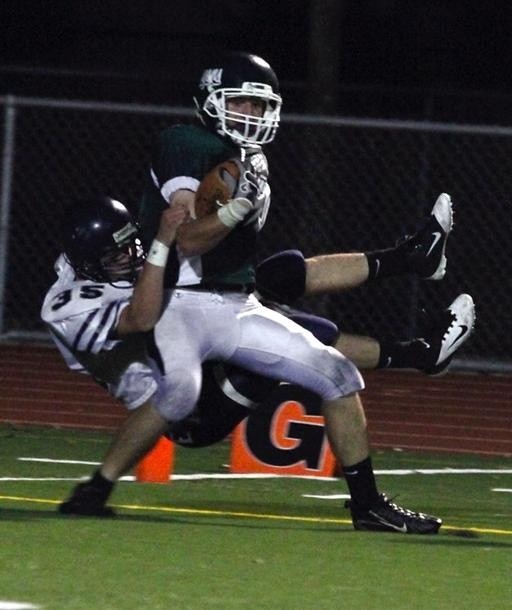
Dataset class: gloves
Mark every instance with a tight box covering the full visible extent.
[219,158,260,229]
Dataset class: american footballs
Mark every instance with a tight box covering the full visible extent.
[196,159,252,222]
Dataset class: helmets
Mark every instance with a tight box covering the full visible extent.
[60,194,146,287]
[194,49,282,141]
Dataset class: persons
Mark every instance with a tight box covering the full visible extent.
[41,193,478,448]
[58,46,442,534]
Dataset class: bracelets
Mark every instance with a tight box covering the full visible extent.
[145,239,169,269]
[217,200,245,226]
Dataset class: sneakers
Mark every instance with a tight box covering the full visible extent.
[58,484,119,521]
[416,293,476,379]
[350,497,443,536]
[408,191,455,285]
